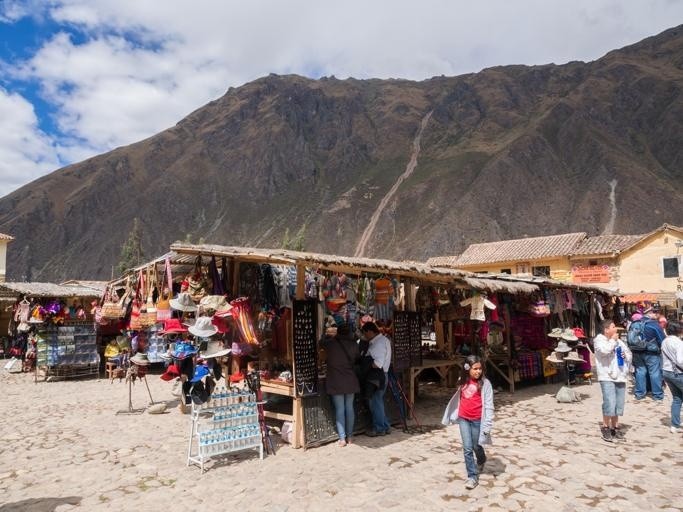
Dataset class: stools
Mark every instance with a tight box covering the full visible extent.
[105,362,117,380]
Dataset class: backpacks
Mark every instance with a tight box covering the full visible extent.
[627,319,655,351]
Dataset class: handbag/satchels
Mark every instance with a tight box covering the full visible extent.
[528,299,550,317]
[555,386,580,402]
[181,272,213,303]
[95,294,173,331]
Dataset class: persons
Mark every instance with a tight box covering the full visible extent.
[321,324,361,448]
[103,338,124,369]
[356,322,393,437]
[442,355,495,489]
[593,307,683,442]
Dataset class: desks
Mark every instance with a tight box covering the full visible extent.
[408,358,463,420]
[259,379,302,449]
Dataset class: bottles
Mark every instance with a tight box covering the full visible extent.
[616,345,623,365]
[201,384,260,455]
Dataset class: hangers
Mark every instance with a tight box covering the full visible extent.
[19,295,30,306]
[192,251,422,286]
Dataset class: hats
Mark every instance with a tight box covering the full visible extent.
[545,328,588,364]
[130,352,151,367]
[644,306,659,313]
[160,294,233,383]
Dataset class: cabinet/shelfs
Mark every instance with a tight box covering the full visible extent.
[33,321,101,381]
[187,388,265,475]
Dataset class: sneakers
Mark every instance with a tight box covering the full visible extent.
[671,426,683,433]
[335,439,352,446]
[367,429,391,436]
[477,456,486,472]
[600,425,626,442]
[466,478,479,489]
